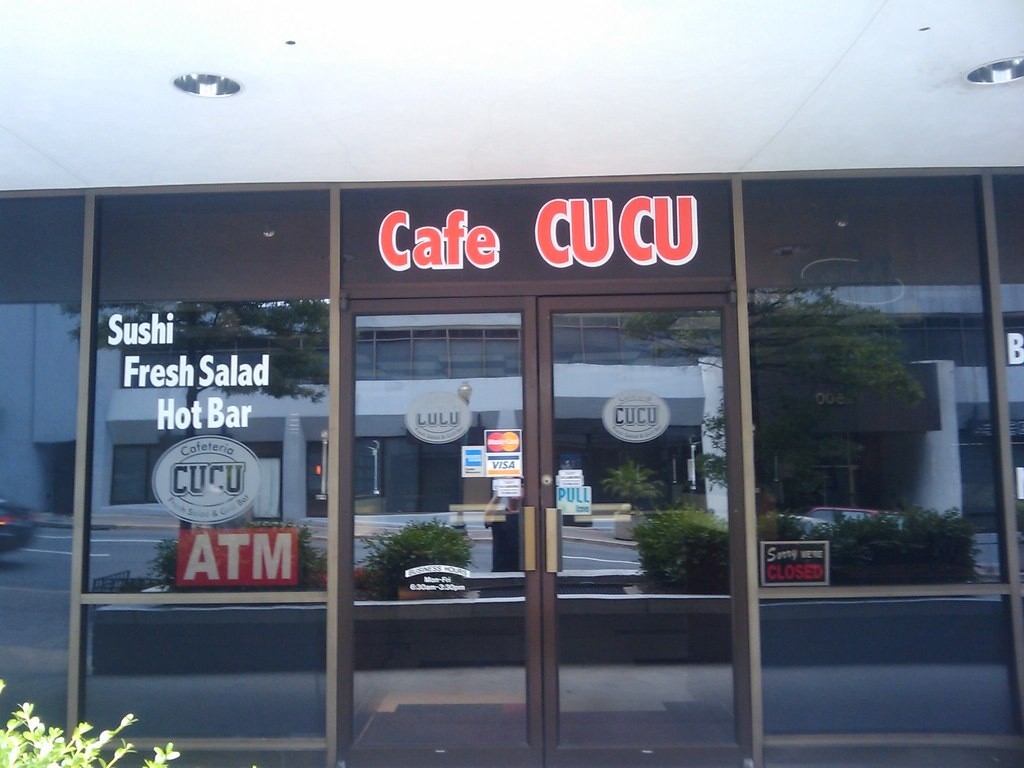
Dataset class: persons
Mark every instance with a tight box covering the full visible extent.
[484,477,525,573]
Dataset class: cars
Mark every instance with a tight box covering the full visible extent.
[973,532,1024,583]
[779,512,832,536]
[0,500,35,552]
[807,508,905,529]
